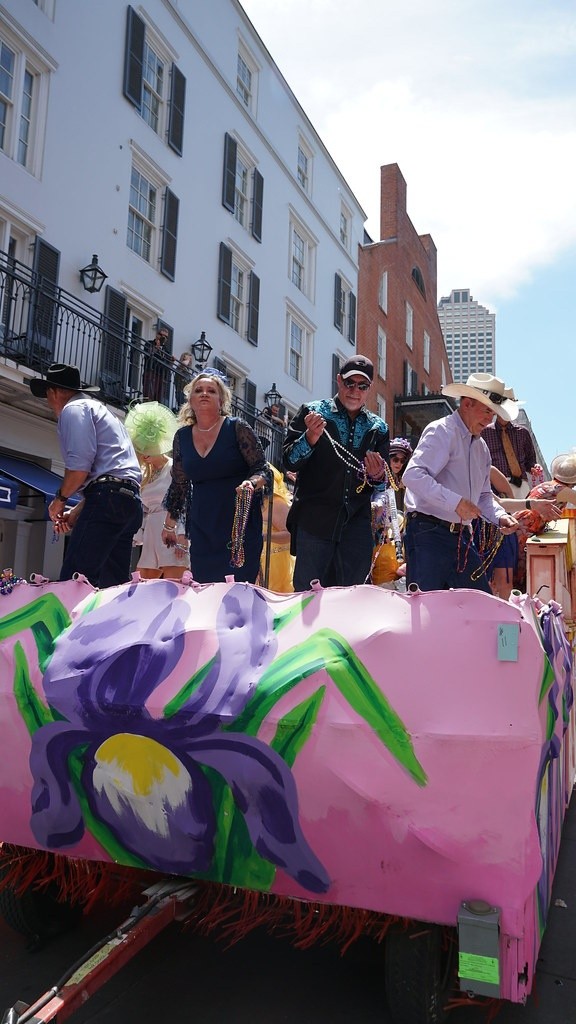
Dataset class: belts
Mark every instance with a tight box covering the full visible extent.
[84,475,137,488]
[410,512,467,534]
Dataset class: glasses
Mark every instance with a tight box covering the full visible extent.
[475,386,507,404]
[342,375,370,391]
[389,453,408,464]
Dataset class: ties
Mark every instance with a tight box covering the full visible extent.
[502,426,522,477]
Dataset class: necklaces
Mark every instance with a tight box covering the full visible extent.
[324,428,399,494]
[198,417,220,432]
[227,487,252,567]
[363,493,389,584]
[457,512,504,581]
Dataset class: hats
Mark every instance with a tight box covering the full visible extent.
[338,355,375,384]
[437,374,521,422]
[504,387,526,406]
[550,454,576,485]
[30,364,104,398]
[388,439,412,456]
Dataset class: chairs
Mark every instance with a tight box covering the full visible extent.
[99,372,143,412]
[1,325,45,370]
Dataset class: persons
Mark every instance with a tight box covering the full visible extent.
[271,403,287,433]
[479,387,537,498]
[173,351,192,410]
[402,372,519,595]
[254,406,272,451]
[389,437,413,512]
[122,396,190,580]
[160,372,274,584]
[490,465,519,600]
[142,328,168,403]
[282,354,390,592]
[30,363,143,589]
[511,446,576,600]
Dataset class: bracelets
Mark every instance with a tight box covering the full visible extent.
[247,479,258,490]
[57,490,68,502]
[163,521,177,532]
[526,498,531,509]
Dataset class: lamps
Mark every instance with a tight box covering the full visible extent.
[264,384,282,408]
[191,332,212,363]
[79,254,107,293]
